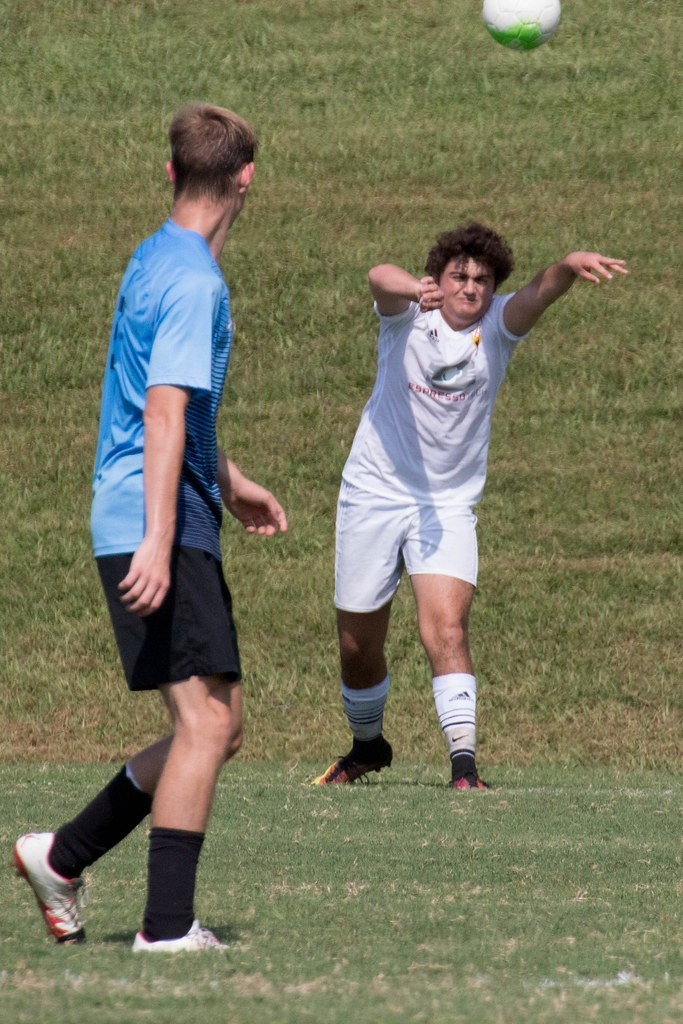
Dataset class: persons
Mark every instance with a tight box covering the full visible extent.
[311,219,630,790]
[13,102,288,956]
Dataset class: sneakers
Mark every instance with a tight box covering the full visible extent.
[132,919,250,955]
[449,771,490,792]
[310,735,392,786]
[12,832,96,945]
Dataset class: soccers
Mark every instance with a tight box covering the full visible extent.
[480,0,562,50]
